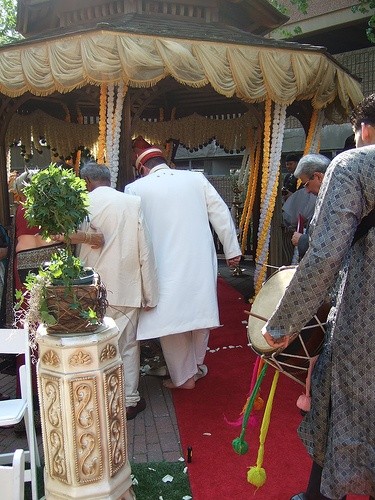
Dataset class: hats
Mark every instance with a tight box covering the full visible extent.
[132,135,164,170]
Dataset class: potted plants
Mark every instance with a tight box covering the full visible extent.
[14,162,108,334]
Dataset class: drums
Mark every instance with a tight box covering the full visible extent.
[247,265,336,388]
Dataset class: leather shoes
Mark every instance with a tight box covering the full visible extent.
[126,398,147,420]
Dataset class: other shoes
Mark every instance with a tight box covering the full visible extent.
[146,366,167,377]
[192,364,208,382]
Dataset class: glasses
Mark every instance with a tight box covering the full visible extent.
[303,181,310,188]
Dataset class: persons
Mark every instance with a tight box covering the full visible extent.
[12,165,105,435]
[261,91,375,426]
[122,138,243,391]
[292,154,344,256]
[280,151,303,199]
[0,214,12,331]
[73,161,157,421]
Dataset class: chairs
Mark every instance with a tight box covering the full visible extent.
[0,325,41,500]
